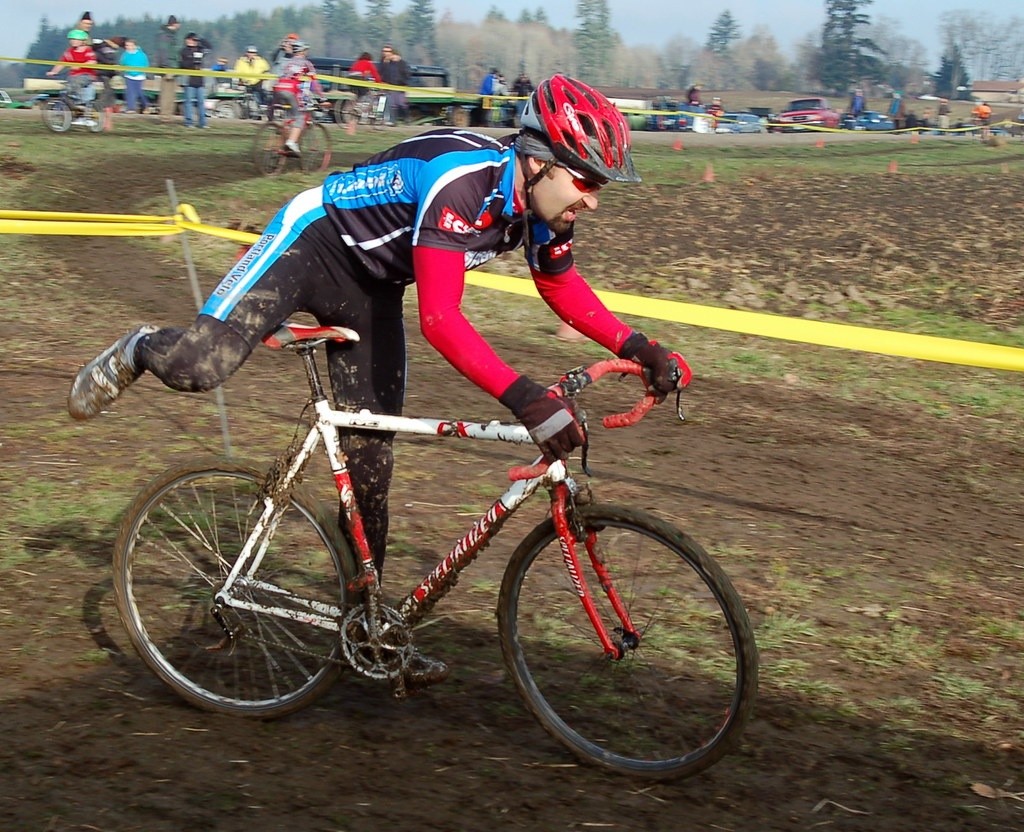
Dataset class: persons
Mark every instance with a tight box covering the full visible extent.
[46,29,96,127]
[349,52,387,118]
[273,42,331,157]
[686,84,704,106]
[179,33,213,129]
[78,12,92,32]
[234,46,270,120]
[271,30,331,107]
[939,99,950,128]
[95,37,126,112]
[888,90,904,117]
[848,88,864,115]
[119,39,150,113]
[482,67,535,96]
[156,15,180,114]
[378,47,394,122]
[972,103,992,125]
[385,52,411,126]
[71,71,695,683]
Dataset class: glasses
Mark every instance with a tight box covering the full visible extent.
[564,165,608,193]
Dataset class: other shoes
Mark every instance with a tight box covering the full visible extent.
[283,140,299,153]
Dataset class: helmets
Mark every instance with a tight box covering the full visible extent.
[66,30,87,40]
[292,42,310,53]
[521,73,643,184]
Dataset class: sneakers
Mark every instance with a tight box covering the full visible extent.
[68,326,161,422]
[343,640,447,685]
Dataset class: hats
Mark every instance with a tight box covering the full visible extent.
[167,15,180,24]
[247,45,258,53]
[184,33,200,41]
[82,11,92,20]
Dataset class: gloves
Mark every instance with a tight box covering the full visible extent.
[498,373,583,463]
[617,330,693,404]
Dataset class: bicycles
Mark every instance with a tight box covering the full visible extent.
[39,70,108,134]
[252,102,334,177]
[109,321,761,782]
[333,85,393,132]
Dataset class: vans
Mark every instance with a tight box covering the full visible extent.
[307,56,453,127]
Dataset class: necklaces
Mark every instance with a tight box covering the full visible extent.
[212,57,232,83]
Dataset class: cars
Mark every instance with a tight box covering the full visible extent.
[771,97,843,133]
[843,110,896,134]
[606,97,763,135]
